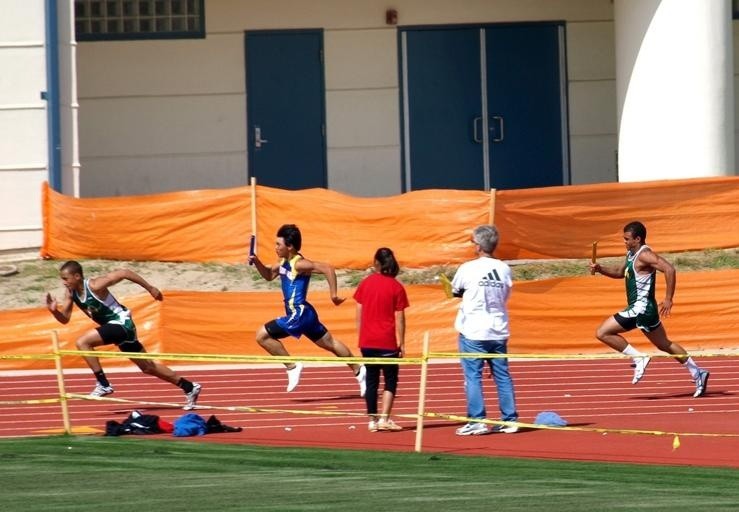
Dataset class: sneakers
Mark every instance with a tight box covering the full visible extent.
[377,419,402,431]
[630,353,651,385]
[90,381,113,397]
[182,383,202,411]
[690,368,709,398]
[355,366,367,397]
[368,418,379,432]
[286,362,303,392]
[455,422,489,436]
[490,424,521,433]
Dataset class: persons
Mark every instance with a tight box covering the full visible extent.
[352,248,410,432]
[248,223,367,398]
[588,220,710,398]
[46,260,201,410]
[450,224,521,436]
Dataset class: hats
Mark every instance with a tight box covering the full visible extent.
[534,411,569,426]
